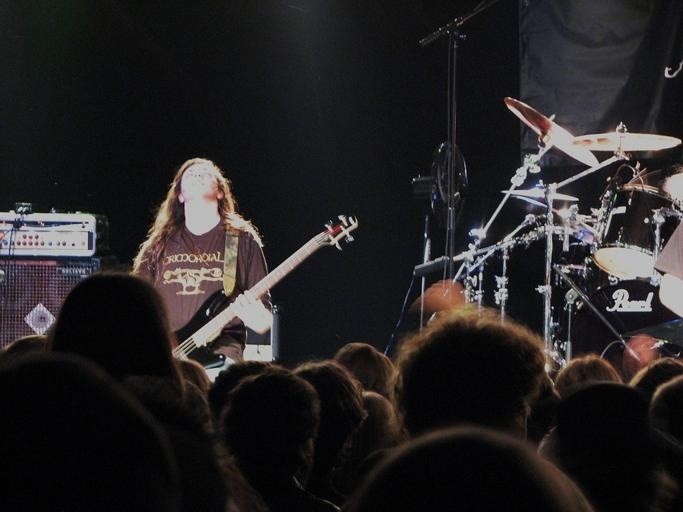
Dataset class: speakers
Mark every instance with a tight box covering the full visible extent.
[0,257,103,349]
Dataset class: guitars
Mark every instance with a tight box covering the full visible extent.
[167,214,359,372]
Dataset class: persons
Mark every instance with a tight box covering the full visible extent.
[655,217,683,320]
[123,154,274,378]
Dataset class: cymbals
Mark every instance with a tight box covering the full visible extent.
[502,188,579,204]
[573,130,683,152]
[504,97,602,167]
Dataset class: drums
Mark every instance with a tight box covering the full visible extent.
[556,241,597,269]
[555,276,683,362]
[592,184,682,279]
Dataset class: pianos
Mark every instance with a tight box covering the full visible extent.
[0,256,116,275]
